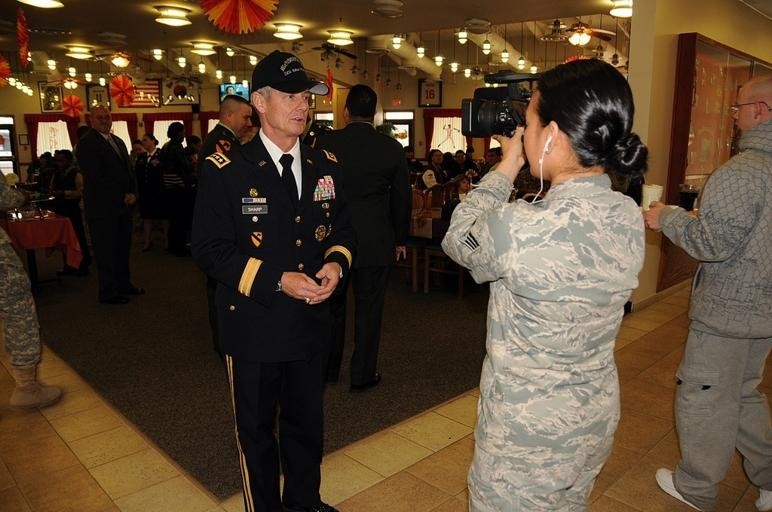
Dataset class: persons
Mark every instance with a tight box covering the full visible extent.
[440,59,654,511]
[15,149,86,260]
[202,95,252,156]
[158,121,197,259]
[402,145,501,202]
[132,139,144,154]
[641,74,772,511]
[320,82,414,393]
[139,132,169,253]
[72,103,147,309]
[0,167,64,413]
[199,49,354,511]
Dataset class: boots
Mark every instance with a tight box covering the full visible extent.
[10,367,62,409]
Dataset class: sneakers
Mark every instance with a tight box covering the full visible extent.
[754,487,771,512]
[655,468,706,510]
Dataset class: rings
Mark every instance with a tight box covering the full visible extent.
[305,297,311,303]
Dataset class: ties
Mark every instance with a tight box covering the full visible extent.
[278,153,300,209]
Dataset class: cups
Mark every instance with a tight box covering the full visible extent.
[642,184,663,211]
[678,184,699,211]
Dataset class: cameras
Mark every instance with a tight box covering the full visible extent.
[462,69,538,138]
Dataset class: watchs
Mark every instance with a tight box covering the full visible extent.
[339,265,344,279]
[275,279,282,293]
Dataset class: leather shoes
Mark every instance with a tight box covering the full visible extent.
[122,285,144,295]
[100,293,130,304]
[313,503,336,512]
[351,372,380,390]
[326,369,338,382]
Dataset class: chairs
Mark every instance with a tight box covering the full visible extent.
[423,238,465,292]
[425,183,465,218]
[396,246,420,292]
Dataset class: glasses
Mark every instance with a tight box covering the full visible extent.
[728,100,772,115]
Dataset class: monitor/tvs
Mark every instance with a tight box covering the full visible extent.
[219,82,251,103]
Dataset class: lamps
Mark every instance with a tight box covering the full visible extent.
[273,22,304,41]
[230,55,237,85]
[566,15,593,46]
[500,23,509,64]
[47,53,57,71]
[65,45,95,61]
[111,51,130,68]
[392,34,405,50]
[625,24,630,70]
[597,13,603,58]
[481,34,490,55]
[518,22,525,70]
[453,27,468,45]
[369,1,404,18]
[198,55,206,74]
[327,29,354,46]
[85,62,93,81]
[432,30,445,68]
[530,21,537,75]
[466,17,491,35]
[100,61,106,87]
[68,58,77,78]
[612,18,619,66]
[610,0,634,19]
[216,55,223,80]
[464,42,471,79]
[417,32,425,60]
[153,5,193,28]
[450,27,459,72]
[19,0,64,9]
[178,48,187,68]
[98,31,128,49]
[191,42,217,56]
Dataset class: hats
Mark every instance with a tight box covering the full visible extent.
[250,50,329,97]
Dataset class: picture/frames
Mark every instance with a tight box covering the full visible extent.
[85,82,112,112]
[418,77,442,107]
[19,133,29,145]
[38,79,65,114]
[117,77,162,109]
[161,77,201,106]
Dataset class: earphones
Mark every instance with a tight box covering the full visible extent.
[534,134,553,203]
